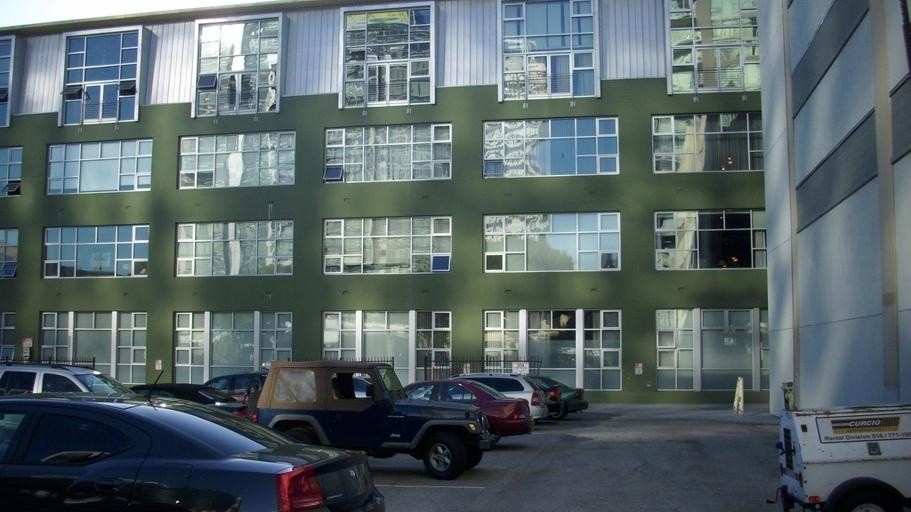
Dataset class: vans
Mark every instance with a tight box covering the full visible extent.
[202,372,258,390]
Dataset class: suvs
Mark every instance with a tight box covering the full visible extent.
[252,359,497,481]
[0,358,140,402]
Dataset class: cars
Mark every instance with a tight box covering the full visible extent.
[404,371,589,444]
[0,396,386,512]
[128,380,248,421]
